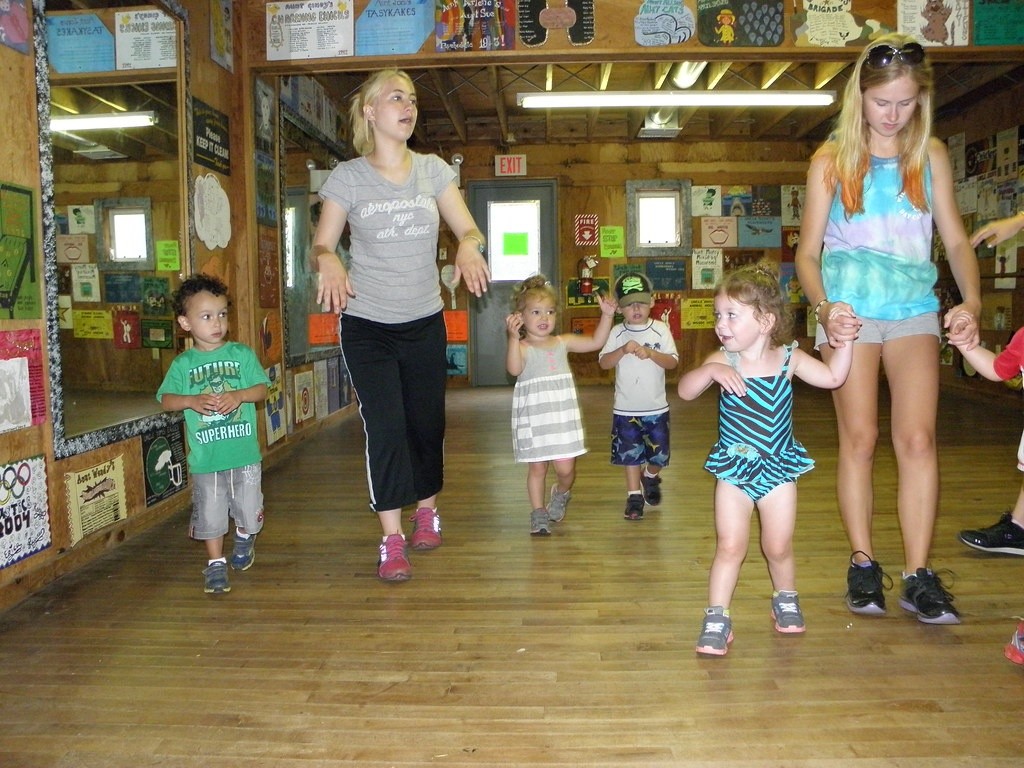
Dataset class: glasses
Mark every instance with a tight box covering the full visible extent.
[860,43,927,70]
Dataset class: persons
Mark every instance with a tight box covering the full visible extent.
[660,305,672,329]
[958,209,1024,554]
[309,67,493,582]
[504,272,619,536]
[154,272,272,595]
[792,27,981,627]
[677,255,856,657]
[597,272,679,519]
[949,308,1024,667]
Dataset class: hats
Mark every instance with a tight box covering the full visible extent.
[613,271,655,307]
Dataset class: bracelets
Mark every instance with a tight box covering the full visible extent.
[463,235,484,252]
[815,299,828,323]
[1018,210,1024,231]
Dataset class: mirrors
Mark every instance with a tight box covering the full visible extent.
[32,0,198,461]
[279,101,353,368]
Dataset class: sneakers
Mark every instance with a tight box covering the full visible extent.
[378,534,412,582]
[623,493,645,520]
[898,566,962,624]
[409,507,442,551]
[844,550,894,615]
[641,466,662,507]
[695,607,735,656]
[201,559,231,594]
[230,532,258,571]
[1003,621,1024,665]
[956,510,1024,556]
[770,590,806,633]
[546,483,570,522]
[531,506,551,534]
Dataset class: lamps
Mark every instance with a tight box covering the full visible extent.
[515,90,837,109]
[448,153,465,187]
[305,157,339,193]
[48,109,160,131]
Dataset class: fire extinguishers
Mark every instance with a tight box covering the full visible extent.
[577,254,598,296]
